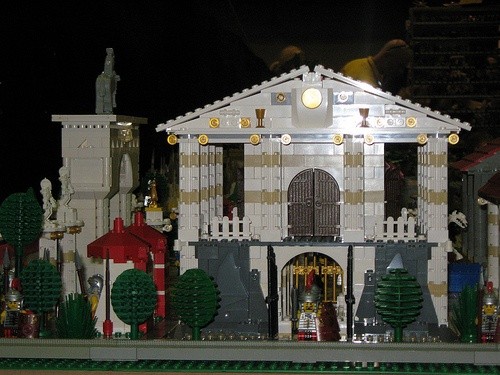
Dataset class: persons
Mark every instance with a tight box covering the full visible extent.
[294,269,321,341]
[481,281,500,343]
[1,278,24,339]
